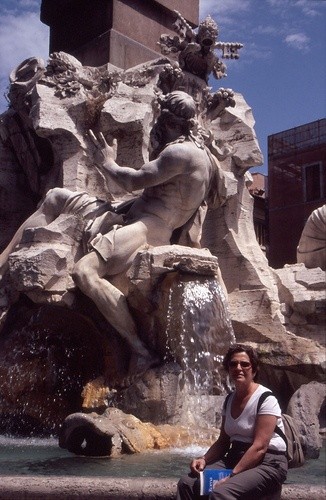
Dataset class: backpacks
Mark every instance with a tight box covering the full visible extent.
[222,391,305,469]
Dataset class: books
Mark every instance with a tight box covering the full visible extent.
[200,468,232,496]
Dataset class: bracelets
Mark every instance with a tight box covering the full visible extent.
[197,456,208,465]
[229,471,237,477]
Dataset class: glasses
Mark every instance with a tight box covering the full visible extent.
[226,361,253,368]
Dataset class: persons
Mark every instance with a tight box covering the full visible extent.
[177,344,288,500]
[0,90,215,389]
